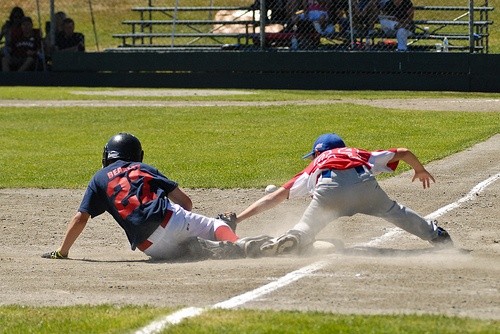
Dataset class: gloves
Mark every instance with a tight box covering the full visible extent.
[42,249,68,259]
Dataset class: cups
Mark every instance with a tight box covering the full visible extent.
[424,27,430,34]
[435,43,442,52]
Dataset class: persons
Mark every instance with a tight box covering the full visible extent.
[215,132,454,256]
[0,7,85,72]
[250,0,416,51]
[40,132,274,262]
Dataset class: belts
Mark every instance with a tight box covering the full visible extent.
[322,165,365,177]
[137,210,173,252]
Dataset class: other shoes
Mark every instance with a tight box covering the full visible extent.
[238,235,274,256]
[189,237,241,260]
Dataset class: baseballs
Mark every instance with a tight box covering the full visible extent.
[265,185,277,193]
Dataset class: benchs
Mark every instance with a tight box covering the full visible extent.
[104,5,495,52]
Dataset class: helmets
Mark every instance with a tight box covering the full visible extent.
[103,133,144,168]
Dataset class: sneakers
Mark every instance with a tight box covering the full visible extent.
[427,227,453,246]
[260,233,300,256]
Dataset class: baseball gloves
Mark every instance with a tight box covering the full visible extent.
[215,212,237,235]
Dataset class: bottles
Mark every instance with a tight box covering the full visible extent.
[396,25,407,50]
[442,37,449,52]
[291,35,297,51]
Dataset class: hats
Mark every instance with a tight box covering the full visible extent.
[302,133,346,158]
